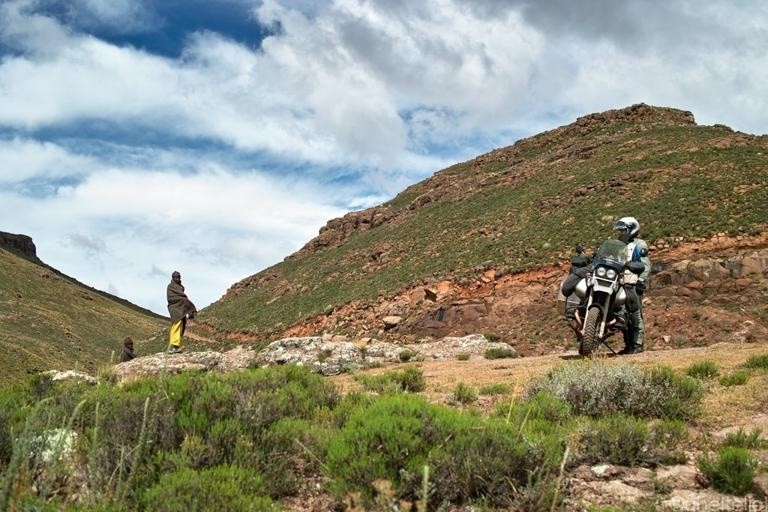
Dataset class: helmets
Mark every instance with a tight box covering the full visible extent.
[612,216,640,243]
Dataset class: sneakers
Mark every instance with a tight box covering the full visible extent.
[617,344,644,355]
[167,346,182,353]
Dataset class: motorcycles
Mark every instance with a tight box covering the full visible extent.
[565,239,648,356]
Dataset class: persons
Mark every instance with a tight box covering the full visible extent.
[606,215,653,355]
[165,269,197,355]
[119,336,136,364]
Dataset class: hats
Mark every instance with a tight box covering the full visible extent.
[172,271,180,277]
[124,337,133,345]
